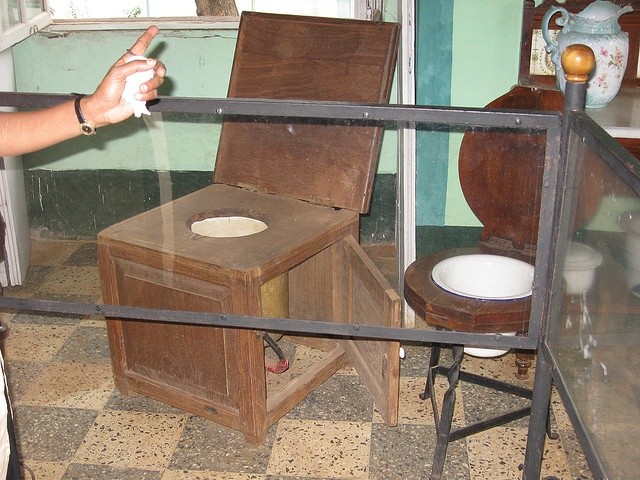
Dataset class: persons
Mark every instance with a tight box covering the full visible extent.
[1,26,166,480]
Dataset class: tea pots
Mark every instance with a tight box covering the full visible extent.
[542,0,634,110]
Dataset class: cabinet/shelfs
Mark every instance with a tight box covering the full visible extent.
[95,10,403,448]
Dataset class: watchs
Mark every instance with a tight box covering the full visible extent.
[73,94,95,138]
